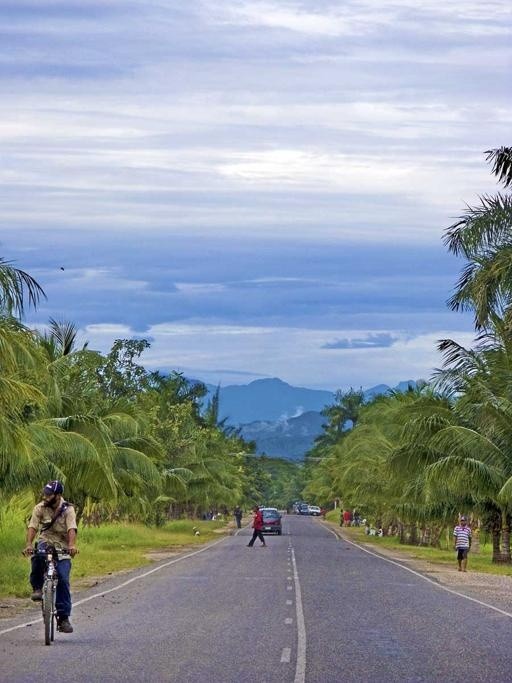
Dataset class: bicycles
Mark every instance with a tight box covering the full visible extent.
[22,547,80,645]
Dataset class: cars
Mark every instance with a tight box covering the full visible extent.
[293,501,321,515]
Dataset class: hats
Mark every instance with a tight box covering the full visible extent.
[43,480,62,496]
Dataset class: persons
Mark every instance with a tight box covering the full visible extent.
[234,505,244,528]
[339,508,360,526]
[452,516,472,572]
[245,505,267,547]
[21,479,79,634]
[223,506,229,520]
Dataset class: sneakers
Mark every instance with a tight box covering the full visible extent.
[31,587,42,601]
[58,615,73,633]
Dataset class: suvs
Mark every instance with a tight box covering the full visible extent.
[256,507,282,535]
